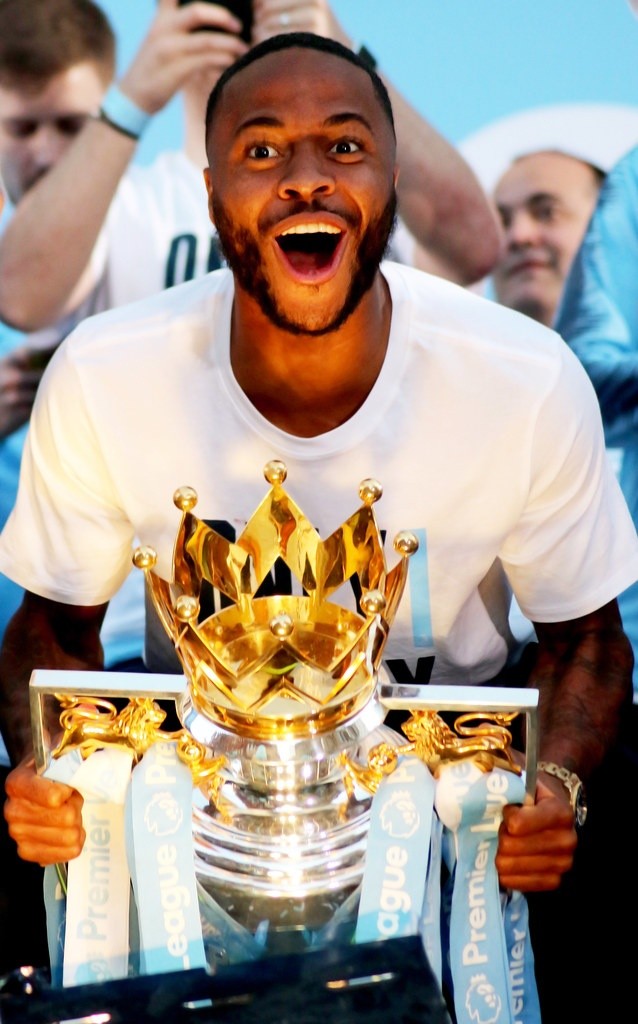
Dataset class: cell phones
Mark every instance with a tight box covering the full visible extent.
[179,0,253,45]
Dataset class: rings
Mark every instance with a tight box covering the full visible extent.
[278,9,291,27]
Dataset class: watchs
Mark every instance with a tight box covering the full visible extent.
[535,760,589,826]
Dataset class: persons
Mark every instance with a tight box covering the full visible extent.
[0,1,637,895]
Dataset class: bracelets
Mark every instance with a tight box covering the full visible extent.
[102,84,154,134]
[97,105,138,140]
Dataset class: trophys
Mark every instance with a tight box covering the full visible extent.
[47,452,538,958]
